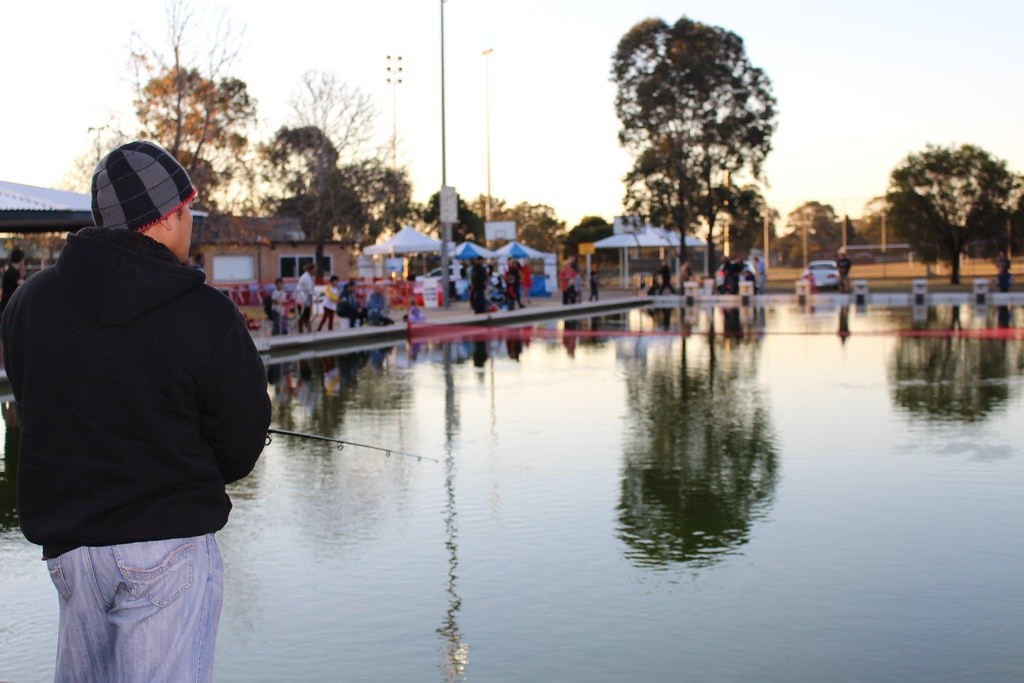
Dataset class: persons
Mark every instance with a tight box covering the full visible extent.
[187,252,205,273]
[408,299,425,321]
[995,252,1012,292]
[317,276,340,331]
[834,252,851,294]
[719,256,768,294]
[657,261,676,295]
[457,256,531,314]
[559,261,582,306]
[0,249,26,316]
[266,278,289,335]
[680,259,695,294]
[338,281,394,329]
[290,264,317,331]
[589,269,600,302]
[0,140,271,683]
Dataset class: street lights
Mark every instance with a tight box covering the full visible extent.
[481,47,495,268]
[383,54,404,277]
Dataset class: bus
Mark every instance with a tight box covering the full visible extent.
[837,244,919,263]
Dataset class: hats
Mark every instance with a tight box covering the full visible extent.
[90,141,196,233]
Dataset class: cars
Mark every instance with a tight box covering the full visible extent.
[803,260,841,292]
[713,257,761,294]
[414,263,467,283]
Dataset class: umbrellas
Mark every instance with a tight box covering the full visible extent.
[492,243,551,261]
[593,226,709,290]
[362,229,457,256]
[446,239,498,261]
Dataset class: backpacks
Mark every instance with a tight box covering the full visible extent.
[264,296,274,320]
